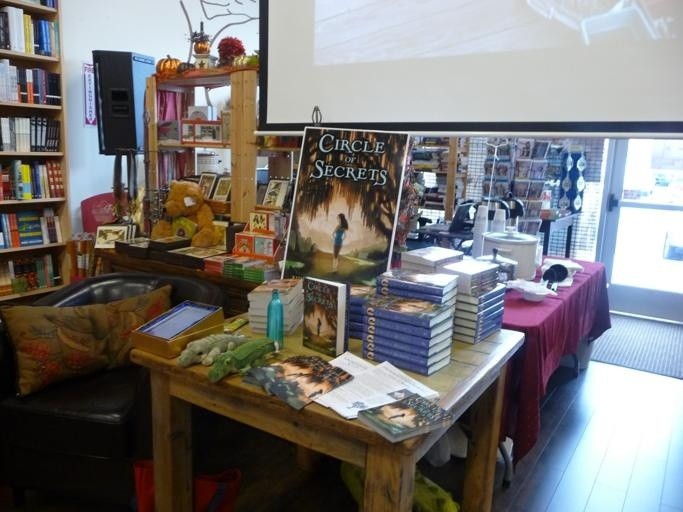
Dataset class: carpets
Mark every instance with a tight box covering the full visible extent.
[589,312,683,380]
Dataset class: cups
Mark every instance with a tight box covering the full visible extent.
[471,204,489,258]
[490,209,506,234]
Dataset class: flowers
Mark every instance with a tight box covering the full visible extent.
[218,37,244,62]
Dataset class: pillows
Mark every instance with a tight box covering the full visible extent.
[0,283,174,398]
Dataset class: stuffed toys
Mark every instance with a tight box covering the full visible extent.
[179,332,279,385]
[148,179,224,249]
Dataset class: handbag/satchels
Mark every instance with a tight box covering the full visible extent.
[132,455,243,512]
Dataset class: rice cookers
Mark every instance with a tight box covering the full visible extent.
[480,226,539,281]
[476,247,517,292]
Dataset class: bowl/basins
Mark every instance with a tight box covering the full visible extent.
[516,283,550,304]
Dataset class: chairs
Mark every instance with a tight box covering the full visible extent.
[1,271,225,507]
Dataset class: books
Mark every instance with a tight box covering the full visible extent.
[206,254,508,443]
[412,137,466,206]
[0,1,106,294]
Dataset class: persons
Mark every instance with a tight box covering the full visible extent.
[252,214,267,228]
[330,213,350,274]
[483,139,544,232]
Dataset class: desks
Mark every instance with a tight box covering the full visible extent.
[502,256,611,490]
[539,213,579,259]
[416,223,473,254]
[131,308,526,512]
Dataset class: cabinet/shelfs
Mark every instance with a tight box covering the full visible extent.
[411,135,469,222]
[0,0,78,304]
[145,64,256,236]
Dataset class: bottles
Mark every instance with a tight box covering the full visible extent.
[266,289,285,351]
